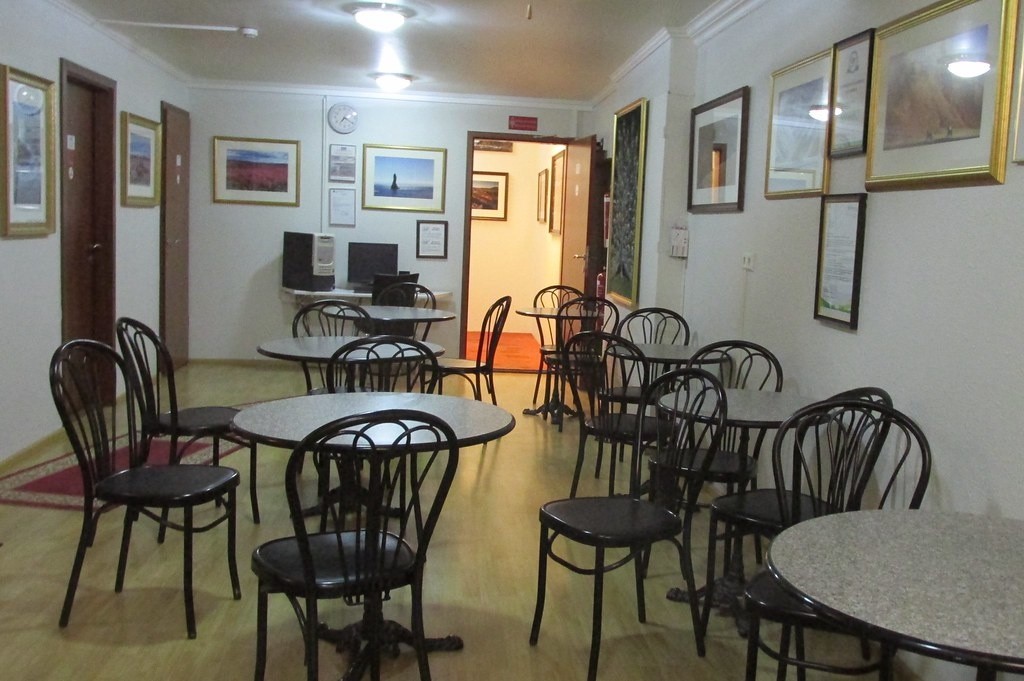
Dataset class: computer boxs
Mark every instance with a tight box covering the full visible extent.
[283,231,335,291]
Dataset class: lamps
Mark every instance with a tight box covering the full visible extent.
[340,4,413,91]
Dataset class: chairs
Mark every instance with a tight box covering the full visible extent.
[252,410,460,681]
[531,286,932,681]
[49,317,260,640]
[294,282,513,516]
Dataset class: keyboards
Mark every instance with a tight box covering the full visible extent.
[353,287,373,293]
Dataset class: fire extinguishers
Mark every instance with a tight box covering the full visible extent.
[595,266,606,331]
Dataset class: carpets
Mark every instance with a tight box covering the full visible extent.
[0,431,244,511]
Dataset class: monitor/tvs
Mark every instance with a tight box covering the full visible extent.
[347,242,398,286]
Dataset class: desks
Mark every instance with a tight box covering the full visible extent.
[658,389,835,628]
[766,509,1024,681]
[516,308,605,424]
[247,306,515,681]
[607,342,726,513]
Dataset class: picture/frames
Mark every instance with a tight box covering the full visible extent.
[765,0,1024,197]
[121,111,162,207]
[1,65,56,238]
[472,140,564,234]
[605,98,647,305]
[213,137,448,212]
[686,86,749,213]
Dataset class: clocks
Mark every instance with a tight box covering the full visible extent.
[327,103,358,134]
